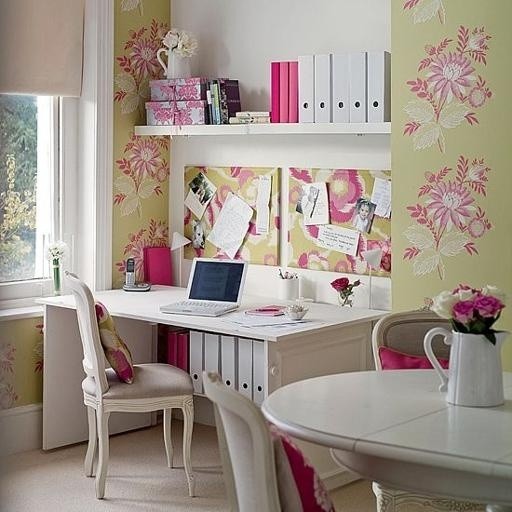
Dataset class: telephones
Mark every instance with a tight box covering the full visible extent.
[123,259,151,291]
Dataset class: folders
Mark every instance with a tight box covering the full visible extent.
[298,51,390,123]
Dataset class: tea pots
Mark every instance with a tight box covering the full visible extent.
[422,325,509,408]
[156,48,194,79]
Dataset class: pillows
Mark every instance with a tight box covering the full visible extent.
[374,342,460,369]
[268,417,340,512]
[93,298,137,387]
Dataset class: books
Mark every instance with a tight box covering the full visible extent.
[245,305,288,317]
[205,79,272,124]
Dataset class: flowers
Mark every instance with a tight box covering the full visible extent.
[425,281,507,343]
[330,274,363,307]
[162,29,201,58]
[44,239,67,292]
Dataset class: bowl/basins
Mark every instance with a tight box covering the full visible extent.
[281,306,308,320]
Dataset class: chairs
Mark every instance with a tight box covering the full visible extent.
[61,267,203,503]
[369,300,508,510]
[201,366,346,511]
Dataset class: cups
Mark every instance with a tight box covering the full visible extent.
[278,278,299,300]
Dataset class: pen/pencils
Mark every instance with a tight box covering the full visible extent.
[278,268,298,279]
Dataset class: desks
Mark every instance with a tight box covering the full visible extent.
[259,364,511,512]
[34,286,389,494]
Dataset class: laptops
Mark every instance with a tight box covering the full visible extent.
[160,257,247,317]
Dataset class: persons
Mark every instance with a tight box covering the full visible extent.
[353,201,370,232]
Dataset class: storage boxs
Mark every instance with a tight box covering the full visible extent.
[145,101,210,125]
[149,78,209,100]
